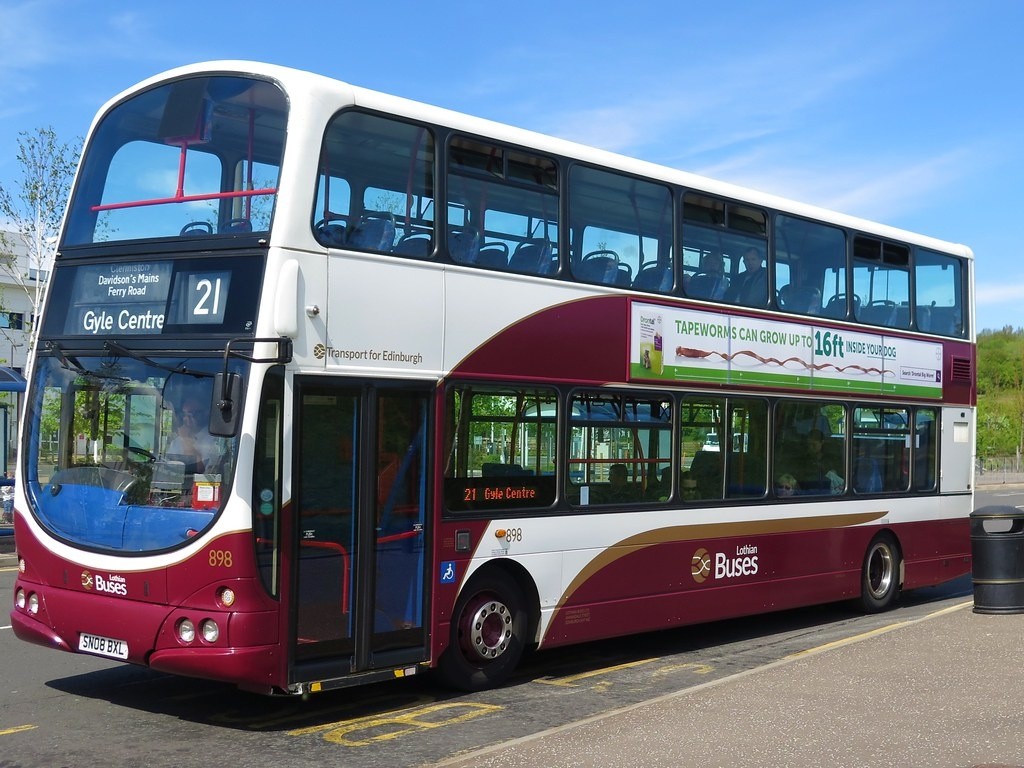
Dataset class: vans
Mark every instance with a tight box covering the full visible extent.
[700,433,748,452]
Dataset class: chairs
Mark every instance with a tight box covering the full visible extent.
[180,211,956,334]
[856,457,880,492]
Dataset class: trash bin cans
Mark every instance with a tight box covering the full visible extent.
[968,505,1024,615]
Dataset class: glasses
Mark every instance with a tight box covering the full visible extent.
[180,410,204,418]
[680,485,698,492]
[777,484,793,490]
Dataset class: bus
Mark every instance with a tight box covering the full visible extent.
[11,59,976,698]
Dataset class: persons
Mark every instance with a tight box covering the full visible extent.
[608,464,633,504]
[777,473,797,497]
[658,468,670,489]
[734,248,766,305]
[1,472,15,518]
[167,395,213,474]
[681,471,703,500]
[684,253,722,297]
[798,429,836,489]
